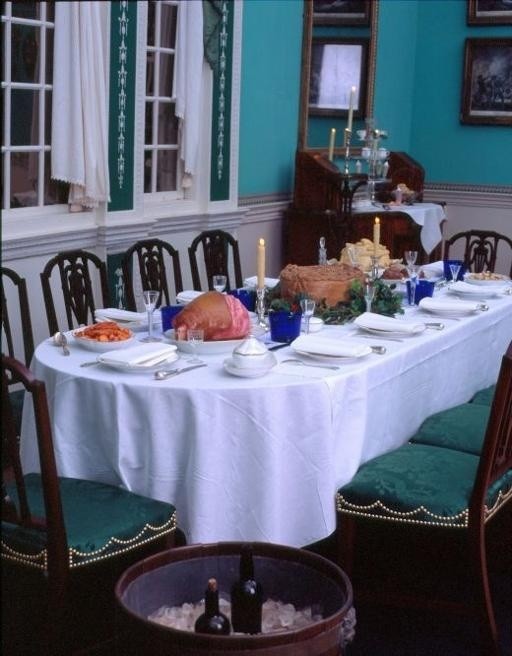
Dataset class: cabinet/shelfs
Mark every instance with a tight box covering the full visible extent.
[277,197,447,272]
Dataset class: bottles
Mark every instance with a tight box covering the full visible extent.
[356,160,388,178]
[396,188,401,205]
[228,543,264,632]
[193,576,231,635]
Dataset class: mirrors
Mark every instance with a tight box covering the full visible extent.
[301,0,379,159]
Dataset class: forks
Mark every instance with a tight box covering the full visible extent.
[282,359,339,371]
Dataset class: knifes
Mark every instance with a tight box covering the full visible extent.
[155,364,206,379]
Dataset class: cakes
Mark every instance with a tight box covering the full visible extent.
[339,238,391,271]
[279,261,368,308]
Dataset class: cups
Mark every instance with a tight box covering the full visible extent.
[212,276,226,293]
[356,128,365,139]
[231,338,273,368]
[360,146,389,157]
[403,250,418,272]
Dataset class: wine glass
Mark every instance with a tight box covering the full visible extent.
[299,298,315,337]
[406,266,422,305]
[140,289,164,342]
[188,329,205,364]
[447,264,462,283]
[346,247,362,266]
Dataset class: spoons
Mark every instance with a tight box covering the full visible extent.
[55,333,70,356]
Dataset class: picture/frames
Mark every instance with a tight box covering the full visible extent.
[308,36,370,120]
[312,0,372,30]
[459,34,512,127]
[466,0,512,27]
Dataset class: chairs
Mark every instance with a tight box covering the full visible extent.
[116,235,186,312]
[332,343,512,655]
[185,228,243,295]
[0,262,38,445]
[0,351,188,654]
[37,247,109,340]
[442,228,511,282]
[411,383,511,455]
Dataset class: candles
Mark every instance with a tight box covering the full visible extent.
[372,216,381,257]
[256,237,267,288]
[354,160,361,174]
[382,160,389,179]
[329,127,334,160]
[347,85,355,129]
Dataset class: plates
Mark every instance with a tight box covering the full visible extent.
[290,333,371,362]
[224,355,278,376]
[71,328,136,351]
[97,351,180,373]
[357,274,510,339]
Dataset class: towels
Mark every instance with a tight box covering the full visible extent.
[175,289,206,303]
[419,260,447,277]
[448,278,504,296]
[243,276,279,289]
[93,307,147,323]
[419,296,481,312]
[290,333,373,358]
[352,310,427,334]
[96,341,177,366]
